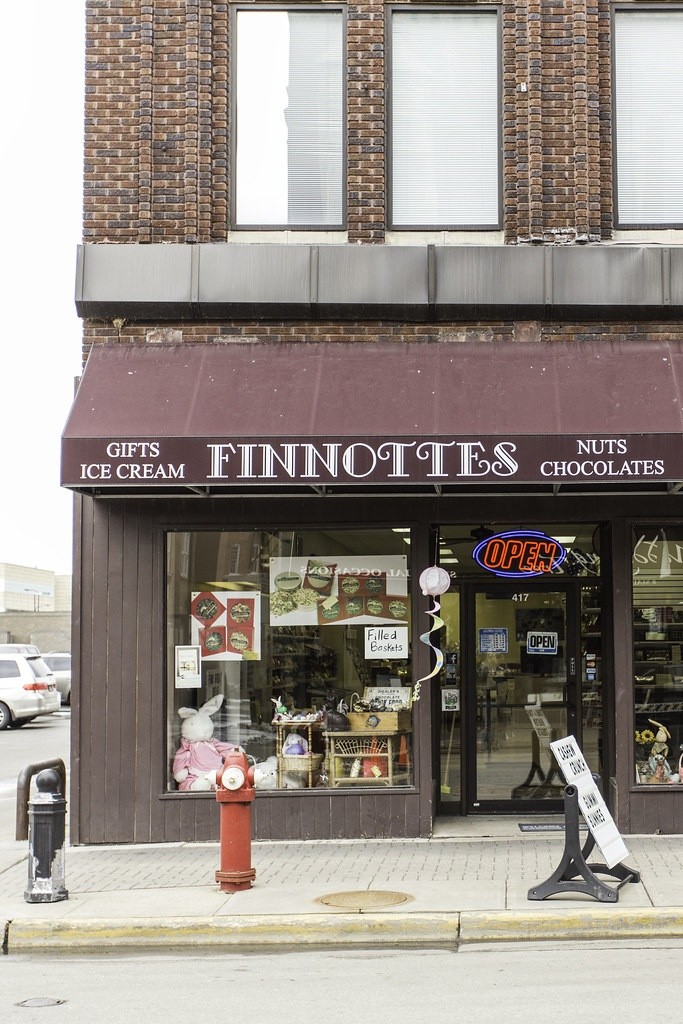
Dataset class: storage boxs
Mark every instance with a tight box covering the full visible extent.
[345,711,412,731]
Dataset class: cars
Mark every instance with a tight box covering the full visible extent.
[38,653,71,706]
[0,654,61,730]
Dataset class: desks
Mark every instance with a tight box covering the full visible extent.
[322,729,412,787]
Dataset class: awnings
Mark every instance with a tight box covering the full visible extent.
[60,342,683,483]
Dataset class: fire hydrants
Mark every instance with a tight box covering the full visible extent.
[215,749,259,891]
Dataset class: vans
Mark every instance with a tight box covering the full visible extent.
[0,644,40,655]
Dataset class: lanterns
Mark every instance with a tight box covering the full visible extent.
[419,568,450,599]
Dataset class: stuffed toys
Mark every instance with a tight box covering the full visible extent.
[173,694,245,791]
[252,734,310,789]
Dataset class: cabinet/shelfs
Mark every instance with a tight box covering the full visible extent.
[241,622,338,725]
[271,722,329,788]
[581,591,683,775]
